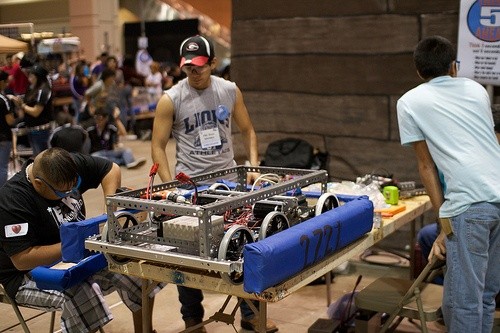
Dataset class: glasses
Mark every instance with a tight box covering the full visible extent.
[34,176,74,197]
[455,60,460,70]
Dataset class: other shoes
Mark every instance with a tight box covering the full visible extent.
[127,157,147,170]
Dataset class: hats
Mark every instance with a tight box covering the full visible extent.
[30,66,48,80]
[179,35,215,68]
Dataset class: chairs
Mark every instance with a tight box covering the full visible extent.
[11,120,57,172]
[0,284,106,333]
[354,255,442,333]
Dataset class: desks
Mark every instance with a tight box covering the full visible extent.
[108,183,433,333]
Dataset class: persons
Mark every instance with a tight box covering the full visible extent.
[135,35,163,93]
[396,35,500,333]
[0,47,190,185]
[152,35,281,333]
[0,146,162,333]
[418,112,500,319]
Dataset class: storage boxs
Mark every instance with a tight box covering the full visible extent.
[354,311,381,333]
